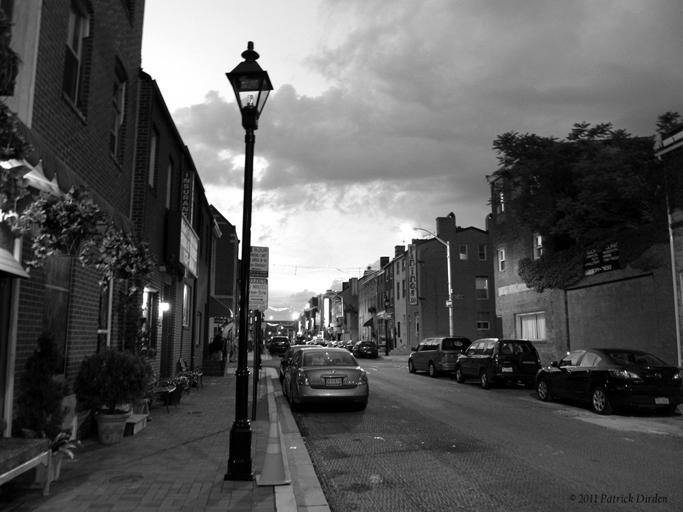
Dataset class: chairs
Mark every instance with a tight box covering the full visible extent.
[147,359,204,415]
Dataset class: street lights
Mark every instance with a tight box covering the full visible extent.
[411,226,456,340]
[224,40,276,481]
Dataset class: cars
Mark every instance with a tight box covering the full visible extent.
[534,347,683,417]
[279,344,369,411]
[265,335,379,359]
[407,336,543,389]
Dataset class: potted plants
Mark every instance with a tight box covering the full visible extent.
[75,345,150,446]
[22,411,84,484]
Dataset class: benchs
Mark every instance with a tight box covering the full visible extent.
[0,438,53,498]
